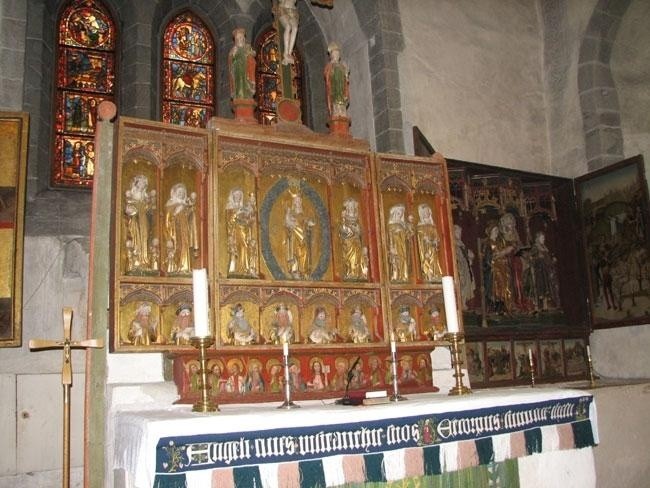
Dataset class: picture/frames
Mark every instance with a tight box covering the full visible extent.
[0,109,31,346]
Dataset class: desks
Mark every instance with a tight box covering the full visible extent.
[125,379,600,487]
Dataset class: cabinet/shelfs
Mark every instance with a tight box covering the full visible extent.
[411,126,650,394]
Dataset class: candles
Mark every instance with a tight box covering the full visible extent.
[528,347,533,359]
[281,331,289,357]
[389,331,396,352]
[186,268,212,339]
[587,344,591,355]
[437,272,461,337]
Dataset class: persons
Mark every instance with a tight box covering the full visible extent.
[227,27,257,98]
[593,205,649,310]
[454,213,562,320]
[277,0,300,65]
[323,42,351,123]
